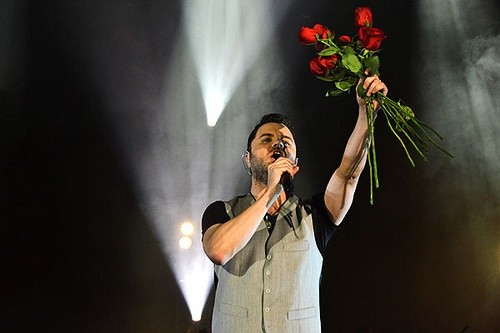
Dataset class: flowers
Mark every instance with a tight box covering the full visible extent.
[298,7,455,204]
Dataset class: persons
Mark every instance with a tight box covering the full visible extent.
[201,68,388,333]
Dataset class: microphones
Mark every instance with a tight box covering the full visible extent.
[281,171,295,198]
